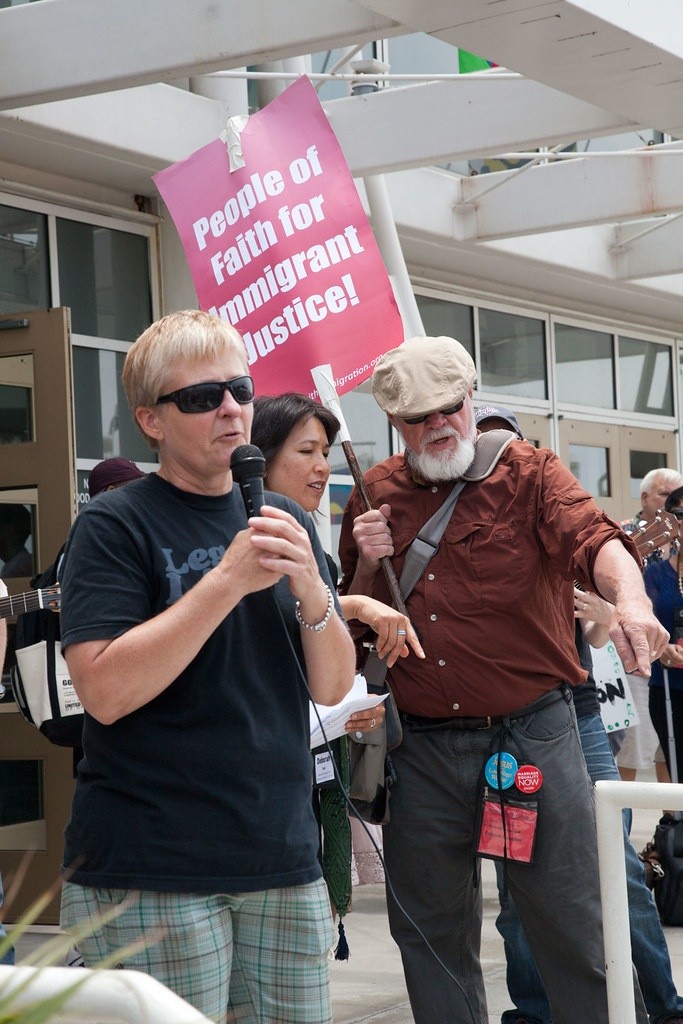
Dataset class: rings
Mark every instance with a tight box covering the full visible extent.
[397,630,406,636]
[667,659,671,665]
[582,603,587,611]
[650,650,658,657]
[370,719,375,729]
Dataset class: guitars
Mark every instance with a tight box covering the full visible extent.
[0,579,62,617]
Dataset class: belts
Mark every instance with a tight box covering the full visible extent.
[404,683,569,732]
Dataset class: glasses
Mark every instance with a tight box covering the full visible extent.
[155,376,256,414]
[403,396,466,424]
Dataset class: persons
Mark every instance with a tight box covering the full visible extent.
[337,336,671,1024]
[56,458,151,966]
[59,309,357,1024]
[250,393,426,962]
[0,579,9,699]
[0,504,33,578]
[474,406,683,1024]
[8,431,28,444]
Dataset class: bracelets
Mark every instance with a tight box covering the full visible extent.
[294,583,335,632]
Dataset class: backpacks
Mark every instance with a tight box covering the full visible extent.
[9,542,84,748]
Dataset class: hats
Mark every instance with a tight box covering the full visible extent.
[372,334,476,416]
[88,458,147,496]
[475,404,523,440]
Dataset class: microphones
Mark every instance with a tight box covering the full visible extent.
[229,446,266,517]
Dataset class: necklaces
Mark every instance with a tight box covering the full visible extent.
[678,557,683,594]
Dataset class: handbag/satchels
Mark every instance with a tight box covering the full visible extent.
[348,671,403,826]
[655,813,683,927]
